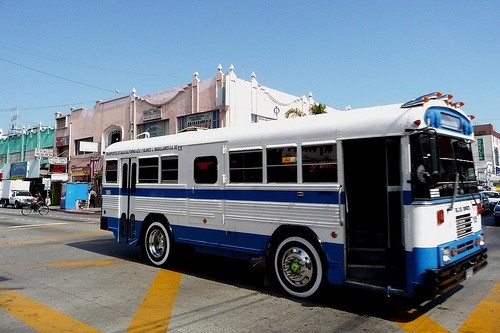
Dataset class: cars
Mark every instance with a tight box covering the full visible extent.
[493,201,500,225]
[480,191,499,214]
[473,193,489,214]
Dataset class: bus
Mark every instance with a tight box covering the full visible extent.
[99,92,489,312]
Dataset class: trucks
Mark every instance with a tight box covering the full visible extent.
[2,180,37,208]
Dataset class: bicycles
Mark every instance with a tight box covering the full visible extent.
[21,199,49,216]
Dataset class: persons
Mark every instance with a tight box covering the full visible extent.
[31,194,38,203]
[32,191,45,210]
[89,188,97,208]
